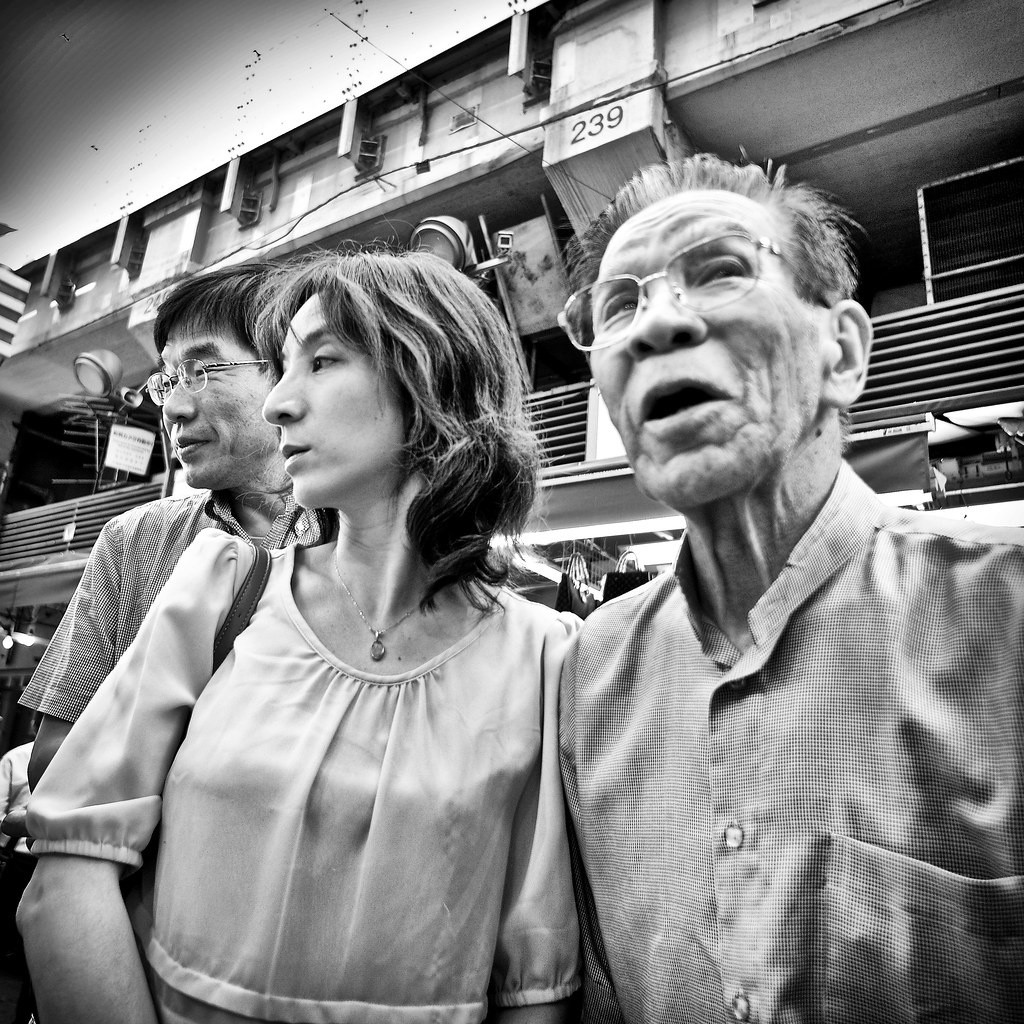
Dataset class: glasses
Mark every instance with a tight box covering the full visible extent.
[144,356,267,406]
[557,231,787,354]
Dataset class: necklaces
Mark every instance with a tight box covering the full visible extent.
[250,536,265,541]
[334,548,421,662]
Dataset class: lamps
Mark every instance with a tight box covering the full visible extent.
[70,345,129,402]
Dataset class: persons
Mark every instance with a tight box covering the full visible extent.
[554,153,1024,1024]
[0,709,42,1024]
[14,263,321,796]
[16,250,584,1024]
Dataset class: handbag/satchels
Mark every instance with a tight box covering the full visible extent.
[553,553,590,618]
[601,551,654,602]
